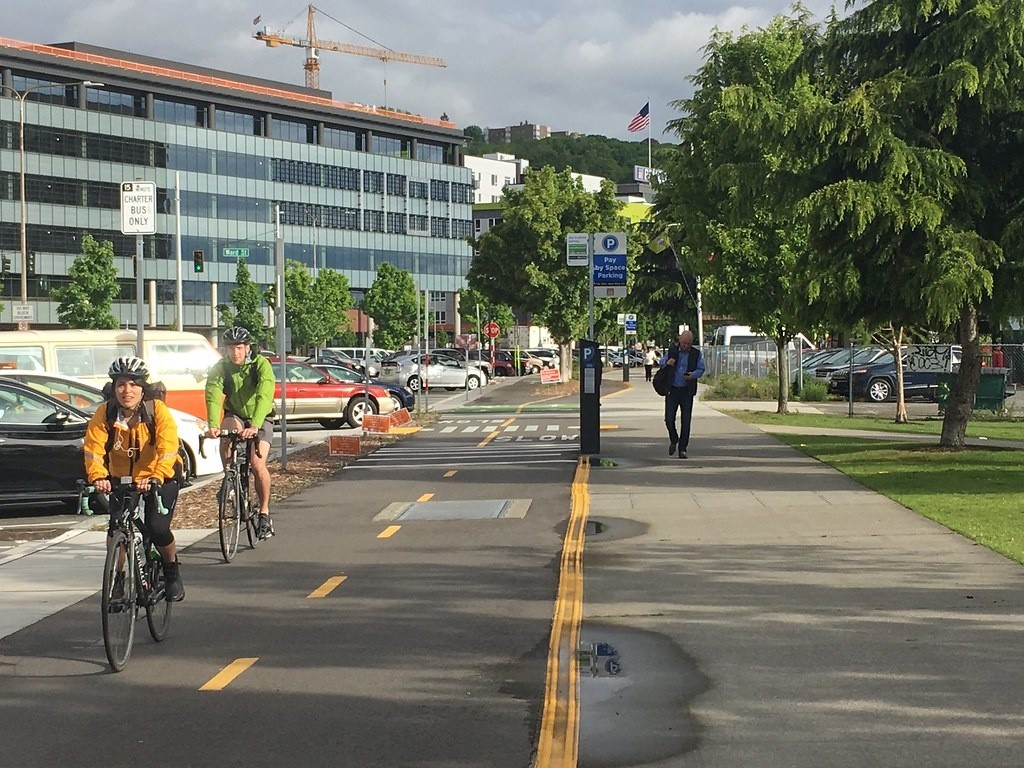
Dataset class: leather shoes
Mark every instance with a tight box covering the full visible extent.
[669,443,676,456]
[679,451,688,459]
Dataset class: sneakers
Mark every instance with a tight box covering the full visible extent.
[256,512,274,539]
[216,487,235,500]
[162,554,184,601]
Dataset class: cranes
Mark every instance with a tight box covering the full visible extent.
[252,4,447,110]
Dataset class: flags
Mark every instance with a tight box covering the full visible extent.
[627,100,651,132]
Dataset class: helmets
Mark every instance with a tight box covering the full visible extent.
[222,326,253,345]
[108,356,149,381]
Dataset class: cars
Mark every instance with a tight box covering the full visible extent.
[322,347,396,376]
[411,347,559,376]
[0,369,224,478]
[711,325,963,402]
[265,356,394,429]
[304,356,365,378]
[378,354,486,391]
[0,377,110,513]
[287,363,415,412]
[572,348,668,367]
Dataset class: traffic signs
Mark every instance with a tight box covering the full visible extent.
[223,248,249,257]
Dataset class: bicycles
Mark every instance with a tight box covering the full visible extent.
[198,429,276,563]
[75,478,182,672]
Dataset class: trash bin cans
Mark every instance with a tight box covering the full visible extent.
[938,373,1017,415]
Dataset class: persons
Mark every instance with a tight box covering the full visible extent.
[642,343,658,382]
[657,330,706,459]
[205,326,275,540]
[84,355,186,613]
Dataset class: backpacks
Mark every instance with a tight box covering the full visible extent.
[101,381,167,444]
[653,343,678,396]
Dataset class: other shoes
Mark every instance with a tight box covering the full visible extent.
[645,378,648,382]
[649,378,651,381]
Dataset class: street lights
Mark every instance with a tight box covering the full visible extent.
[1,81,104,329]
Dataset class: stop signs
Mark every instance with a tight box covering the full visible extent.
[484,323,500,338]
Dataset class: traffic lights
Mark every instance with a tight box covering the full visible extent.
[28,252,35,277]
[2,256,11,277]
[193,251,204,273]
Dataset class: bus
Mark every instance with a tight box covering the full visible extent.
[0,329,226,427]
